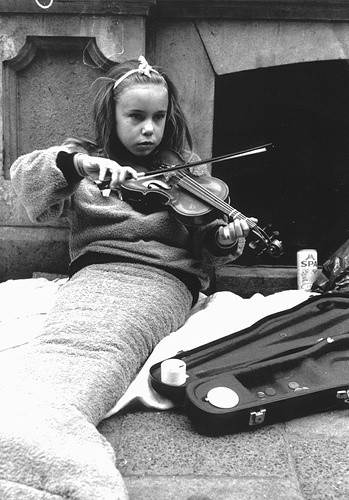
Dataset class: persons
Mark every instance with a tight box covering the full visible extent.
[0,55,260,500]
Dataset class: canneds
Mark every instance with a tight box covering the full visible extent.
[298,248,319,290]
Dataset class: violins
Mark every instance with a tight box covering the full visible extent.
[120,147,284,259]
